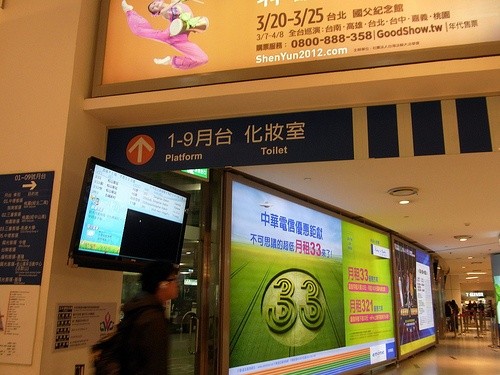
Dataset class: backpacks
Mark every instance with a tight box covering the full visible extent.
[91,304,161,374]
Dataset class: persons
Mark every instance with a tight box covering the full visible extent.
[449,300,459,332]
[95,262,181,375]
[488,299,494,322]
[468,300,485,323]
[119,0,211,69]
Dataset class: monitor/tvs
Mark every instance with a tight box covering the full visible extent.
[66,155,191,278]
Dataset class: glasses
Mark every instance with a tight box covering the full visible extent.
[162,277,176,282]
